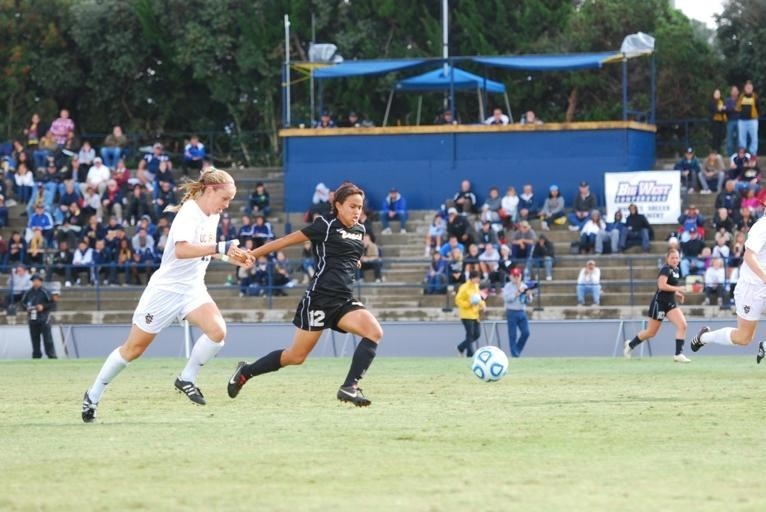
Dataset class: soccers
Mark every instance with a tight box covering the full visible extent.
[471,347,509,385]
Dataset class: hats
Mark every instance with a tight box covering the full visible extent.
[153,142,163,149]
[549,185,560,192]
[30,273,44,281]
[688,204,697,209]
[349,112,358,117]
[688,226,697,234]
[508,267,523,276]
[106,179,117,186]
[389,187,398,193]
[587,260,595,265]
[578,181,590,187]
[318,110,330,117]
[93,157,102,164]
[47,161,56,169]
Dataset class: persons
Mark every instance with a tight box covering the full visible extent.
[503,267,533,358]
[454,271,486,357]
[519,112,543,125]
[228,181,384,406]
[311,112,361,128]
[623,249,691,362]
[691,207,766,364]
[485,108,510,126]
[657,81,766,309]
[82,167,252,424]
[20,274,58,359]
[423,179,656,311]
[1,109,292,325]
[441,111,458,125]
[299,183,408,285]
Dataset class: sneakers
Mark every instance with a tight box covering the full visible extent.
[227,360,252,399]
[81,388,98,424]
[336,382,372,407]
[171,377,208,407]
[756,341,766,365]
[673,354,691,364]
[624,340,634,361]
[690,325,711,352]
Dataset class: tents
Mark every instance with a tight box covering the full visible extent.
[380,64,514,128]
